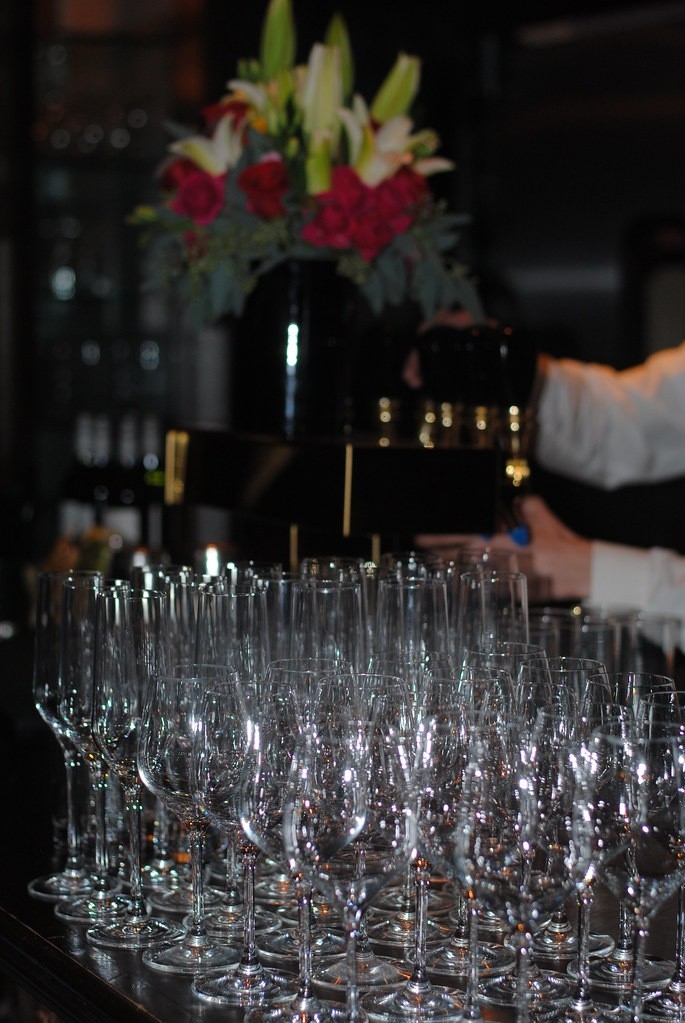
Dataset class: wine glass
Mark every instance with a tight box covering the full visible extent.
[38,557,685,1023]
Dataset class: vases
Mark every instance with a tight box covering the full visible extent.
[226,258,387,572]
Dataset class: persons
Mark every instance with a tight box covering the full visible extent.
[402,309,685,654]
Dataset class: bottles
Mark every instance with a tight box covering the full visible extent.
[346,395,560,545]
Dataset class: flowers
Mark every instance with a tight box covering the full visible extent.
[121,0,487,329]
